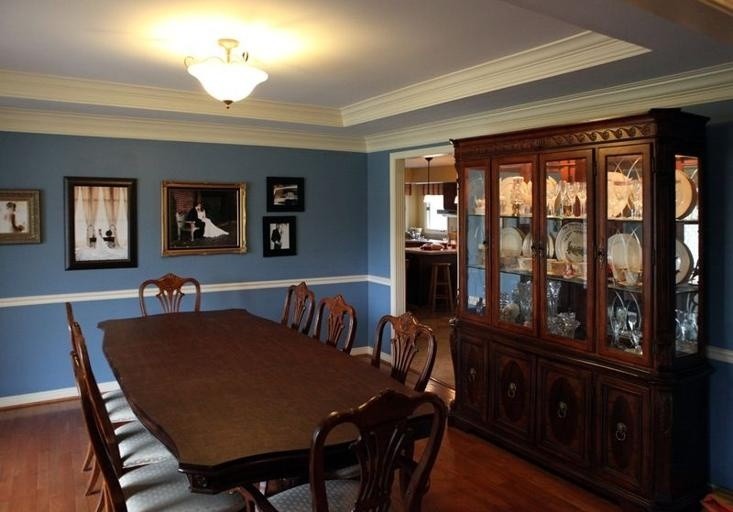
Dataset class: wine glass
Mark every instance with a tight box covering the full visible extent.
[675,310,698,348]
[610,306,642,352]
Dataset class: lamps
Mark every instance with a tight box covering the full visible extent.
[183,36,269,112]
[423,156,435,205]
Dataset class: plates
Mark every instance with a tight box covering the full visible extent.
[498,168,692,287]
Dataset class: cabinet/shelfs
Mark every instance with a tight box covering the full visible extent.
[447,127,537,463]
[537,109,719,512]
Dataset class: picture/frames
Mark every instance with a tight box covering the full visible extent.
[158,179,250,258]
[265,176,306,213]
[63,175,139,272]
[0,188,41,246]
[261,215,297,258]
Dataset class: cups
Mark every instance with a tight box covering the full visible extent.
[498,282,579,339]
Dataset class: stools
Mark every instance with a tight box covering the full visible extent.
[428,262,454,313]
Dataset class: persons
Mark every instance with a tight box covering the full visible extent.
[284,191,298,204]
[186,201,230,239]
[273,183,287,205]
[98,223,120,249]
[270,223,290,249]
[3,201,23,233]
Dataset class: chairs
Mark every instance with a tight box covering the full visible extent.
[280,281,316,338]
[325,311,438,500]
[68,349,248,512]
[63,301,155,497]
[130,271,212,319]
[226,387,447,512]
[59,321,175,512]
[311,293,357,358]
